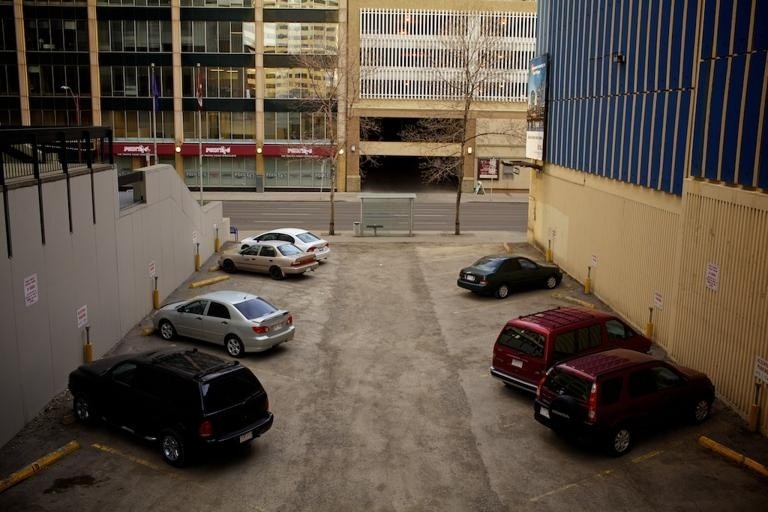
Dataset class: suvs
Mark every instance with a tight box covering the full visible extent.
[67,346,274,469]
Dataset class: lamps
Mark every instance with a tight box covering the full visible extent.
[350,143,356,153]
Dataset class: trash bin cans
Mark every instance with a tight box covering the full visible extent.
[353,222,361,237]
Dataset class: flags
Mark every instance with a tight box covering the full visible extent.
[150,69,159,112]
[193,69,205,114]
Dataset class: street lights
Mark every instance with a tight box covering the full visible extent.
[60,85,81,163]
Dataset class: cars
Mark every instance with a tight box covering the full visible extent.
[151,290,295,357]
[490,305,652,401]
[457,253,562,299]
[217,240,319,281]
[534,348,715,457]
[239,227,332,261]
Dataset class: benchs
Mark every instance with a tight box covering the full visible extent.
[365,225,383,236]
